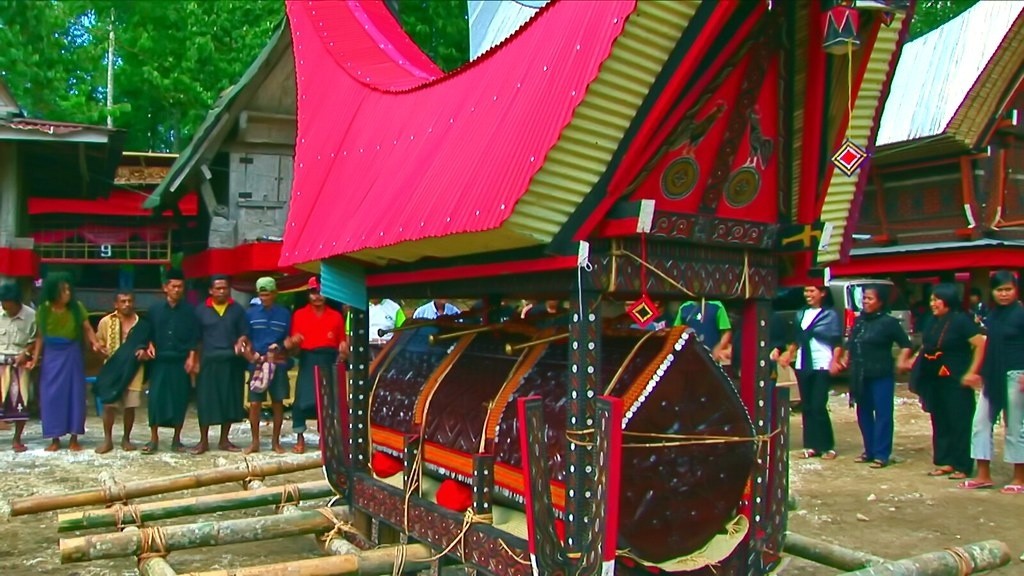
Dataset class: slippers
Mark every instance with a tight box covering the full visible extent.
[293,445,304,453]
[222,444,242,451]
[141,442,157,454]
[12,440,25,452]
[172,443,187,453]
[1001,484,1024,493]
[949,475,969,479]
[956,481,992,489]
[929,469,954,475]
[190,447,210,454]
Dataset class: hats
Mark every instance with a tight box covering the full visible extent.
[308,275,321,291]
[968,288,980,296]
[167,270,184,279]
[255,276,276,292]
[0,283,21,302]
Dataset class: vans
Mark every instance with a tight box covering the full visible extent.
[770,278,914,379]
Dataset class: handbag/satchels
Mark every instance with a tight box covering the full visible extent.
[248,360,275,393]
[775,360,802,401]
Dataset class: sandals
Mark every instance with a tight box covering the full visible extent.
[868,461,886,467]
[797,449,818,458]
[856,453,871,462]
[822,450,837,459]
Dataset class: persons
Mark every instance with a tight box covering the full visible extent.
[0,270,1024,495]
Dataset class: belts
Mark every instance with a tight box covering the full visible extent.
[1006,371,1024,379]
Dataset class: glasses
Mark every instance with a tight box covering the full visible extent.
[996,288,1011,292]
